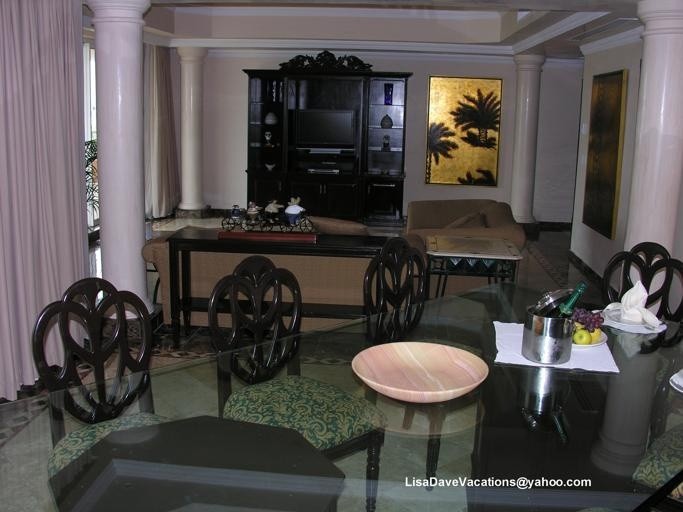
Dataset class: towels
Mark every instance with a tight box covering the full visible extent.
[619,280,659,324]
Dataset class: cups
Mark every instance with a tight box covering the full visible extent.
[525,366,571,418]
[384,83,393,105]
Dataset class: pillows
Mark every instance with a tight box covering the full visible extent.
[304,214,368,238]
[441,210,488,228]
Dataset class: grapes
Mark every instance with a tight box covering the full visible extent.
[571,307,604,331]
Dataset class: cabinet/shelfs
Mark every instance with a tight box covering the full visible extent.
[241,69,287,173]
[247,174,285,207]
[364,71,414,175]
[286,174,366,218]
[366,175,404,223]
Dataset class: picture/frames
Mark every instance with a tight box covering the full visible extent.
[581,69,628,240]
[424,76,502,187]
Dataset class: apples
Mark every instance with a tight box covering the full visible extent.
[574,320,584,330]
[573,330,592,344]
[589,327,601,343]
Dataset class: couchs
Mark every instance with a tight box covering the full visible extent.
[406,198,526,253]
[140,226,427,330]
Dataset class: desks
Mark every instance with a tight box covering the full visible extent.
[166,225,387,356]
[424,234,524,298]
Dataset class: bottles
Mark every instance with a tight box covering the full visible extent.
[383,135,389,147]
[553,280,587,318]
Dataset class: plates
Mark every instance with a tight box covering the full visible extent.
[352,386,486,438]
[604,310,649,328]
[669,373,682,394]
[349,340,492,406]
[572,329,608,350]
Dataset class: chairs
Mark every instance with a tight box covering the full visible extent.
[630,420,683,503]
[205,255,388,512]
[595,241,683,450]
[361,236,489,491]
[29,275,176,484]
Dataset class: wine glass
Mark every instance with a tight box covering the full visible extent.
[265,131,272,144]
